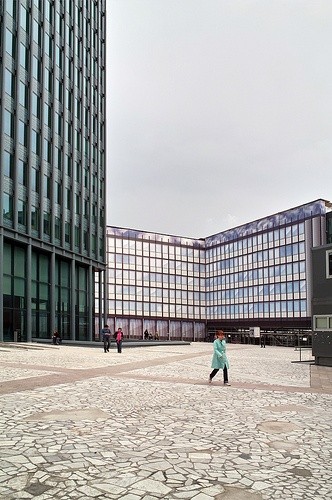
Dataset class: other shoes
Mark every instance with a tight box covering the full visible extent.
[209,374,212,383]
[225,383,231,386]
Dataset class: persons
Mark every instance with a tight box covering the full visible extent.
[51,328,62,345]
[261,336,265,348]
[144,329,159,341]
[113,328,124,353]
[208,329,231,386]
[100,324,112,353]
